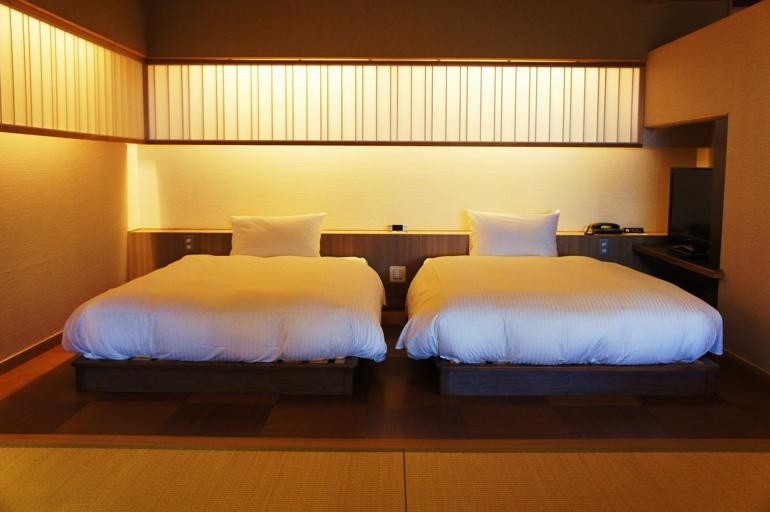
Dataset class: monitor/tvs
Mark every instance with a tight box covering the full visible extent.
[668,166,714,259]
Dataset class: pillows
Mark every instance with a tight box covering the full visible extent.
[466,205,563,259]
[227,214,331,260]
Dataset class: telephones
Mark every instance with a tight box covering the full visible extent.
[591,222,622,234]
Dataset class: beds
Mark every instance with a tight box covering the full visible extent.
[395,254,730,399]
[60,252,393,399]
